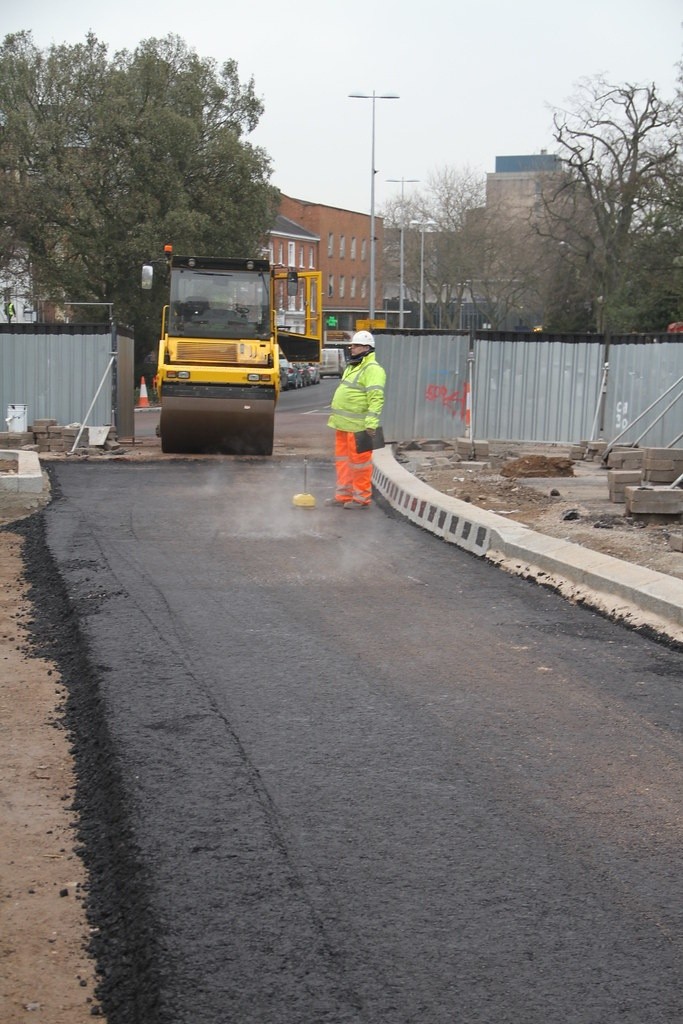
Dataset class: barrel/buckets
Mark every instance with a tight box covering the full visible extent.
[6,404,28,432]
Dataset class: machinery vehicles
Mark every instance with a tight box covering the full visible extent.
[139,243,323,457]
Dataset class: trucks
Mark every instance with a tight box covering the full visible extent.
[319,348,346,378]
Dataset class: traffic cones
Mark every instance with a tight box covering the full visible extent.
[136,376,151,407]
[464,382,471,428]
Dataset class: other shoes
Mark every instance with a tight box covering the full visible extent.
[344,500,369,509]
[325,497,343,507]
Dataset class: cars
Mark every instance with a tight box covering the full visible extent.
[279,359,321,391]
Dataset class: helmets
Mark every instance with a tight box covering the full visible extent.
[351,330,375,348]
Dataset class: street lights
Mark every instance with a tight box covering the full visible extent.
[411,219,437,328]
[386,174,420,329]
[348,89,401,322]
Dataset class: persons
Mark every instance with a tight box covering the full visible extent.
[326,329,385,510]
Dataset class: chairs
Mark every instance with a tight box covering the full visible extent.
[179,296,209,324]
